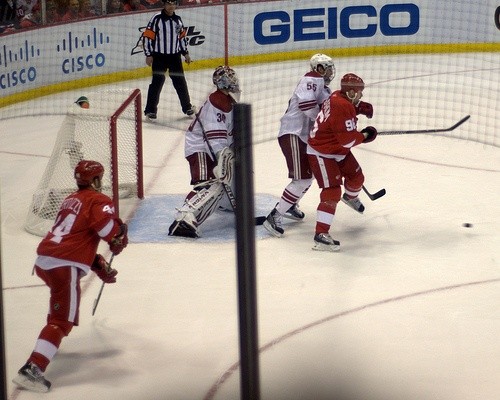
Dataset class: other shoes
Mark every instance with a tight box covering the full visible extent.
[145,111,157,122]
[186,108,195,118]
[168,220,201,239]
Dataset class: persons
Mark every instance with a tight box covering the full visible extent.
[142,0,197,118]
[306,73,379,250]
[168,66,238,238]
[0,0,223,41]
[264,52,337,234]
[13,159,132,393]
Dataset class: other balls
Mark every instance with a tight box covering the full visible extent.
[462,223,473,227]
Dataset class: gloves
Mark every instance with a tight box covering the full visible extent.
[108,222,130,255]
[361,126,378,145]
[355,100,373,120]
[87,254,119,284]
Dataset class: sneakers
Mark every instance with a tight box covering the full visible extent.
[262,208,285,238]
[311,231,341,252]
[285,203,306,218]
[342,193,366,214]
[11,361,51,393]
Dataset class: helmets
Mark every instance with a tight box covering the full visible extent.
[341,73,365,94]
[310,53,336,86]
[212,64,239,94]
[74,161,104,188]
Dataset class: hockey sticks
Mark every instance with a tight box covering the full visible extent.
[377,115,471,134]
[192,104,267,227]
[361,184,386,201]
[92,253,115,316]
